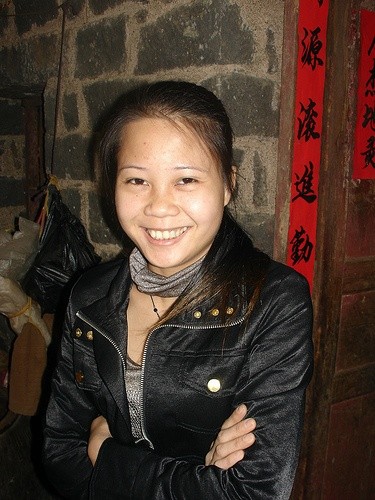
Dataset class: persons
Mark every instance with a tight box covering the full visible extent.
[42,80,314,500]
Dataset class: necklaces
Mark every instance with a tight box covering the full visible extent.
[150,294,161,321]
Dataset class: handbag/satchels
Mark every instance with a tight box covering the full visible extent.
[20,182,102,312]
